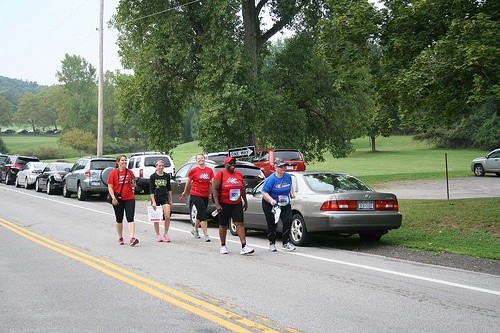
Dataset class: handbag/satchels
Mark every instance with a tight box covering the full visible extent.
[107,191,121,205]
[212,170,223,203]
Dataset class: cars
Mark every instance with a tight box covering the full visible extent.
[470,148,500,178]
[1,128,16,135]
[28,129,40,135]
[14,161,51,190]
[16,129,29,135]
[226,170,403,246]
[44,129,61,134]
[35,162,75,195]
[162,145,307,228]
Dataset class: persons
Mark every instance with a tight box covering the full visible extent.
[261,159,296,252]
[108,155,139,246]
[212,157,254,254]
[181,154,214,242]
[149,160,173,242]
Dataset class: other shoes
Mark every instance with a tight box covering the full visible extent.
[161,234,170,242]
[155,234,163,242]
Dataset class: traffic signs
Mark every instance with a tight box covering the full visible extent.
[228,145,256,158]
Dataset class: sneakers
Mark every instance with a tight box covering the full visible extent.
[281,241,296,251]
[269,243,277,252]
[130,237,139,246]
[118,237,124,244]
[205,235,210,242]
[191,227,201,238]
[220,245,228,254]
[240,245,254,254]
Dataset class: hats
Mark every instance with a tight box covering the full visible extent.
[224,157,236,164]
[274,161,286,167]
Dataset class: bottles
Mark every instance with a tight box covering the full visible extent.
[211,208,222,217]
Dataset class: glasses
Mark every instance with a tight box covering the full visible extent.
[229,162,236,164]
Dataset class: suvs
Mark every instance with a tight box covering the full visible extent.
[0,153,40,185]
[61,155,118,201]
[125,151,177,195]
